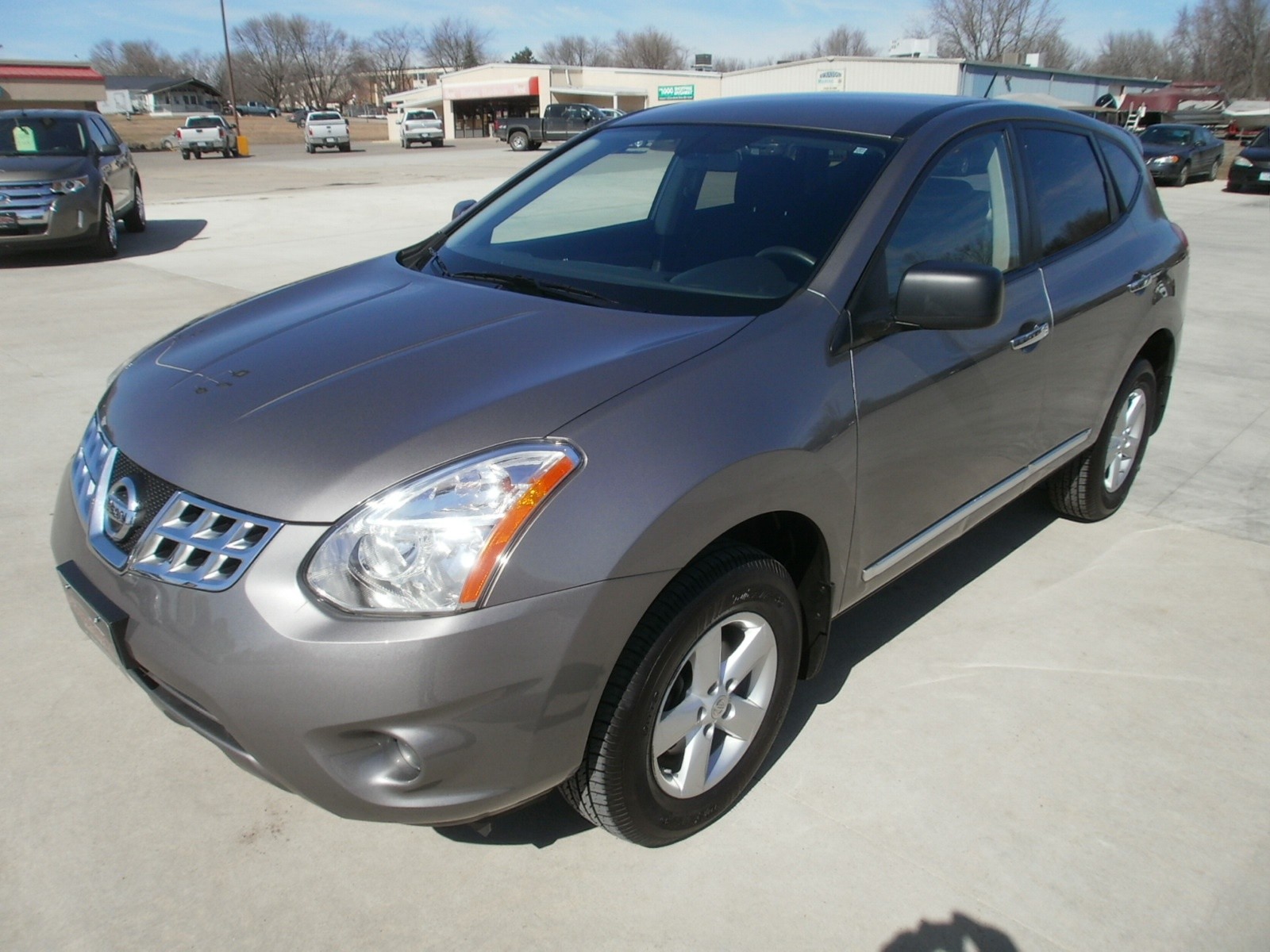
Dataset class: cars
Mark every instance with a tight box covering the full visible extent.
[1126,122,1225,186]
[286,107,342,127]
[51,91,1189,851]
[1228,127,1270,191]
[0,107,146,257]
[160,132,180,151]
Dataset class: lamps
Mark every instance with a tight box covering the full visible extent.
[1005,75,1012,83]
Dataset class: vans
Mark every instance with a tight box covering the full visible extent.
[585,107,648,149]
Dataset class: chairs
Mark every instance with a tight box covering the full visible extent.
[685,154,823,269]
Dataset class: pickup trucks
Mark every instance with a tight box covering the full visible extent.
[303,111,351,154]
[494,103,609,152]
[397,108,445,149]
[236,101,281,118]
[176,115,239,158]
